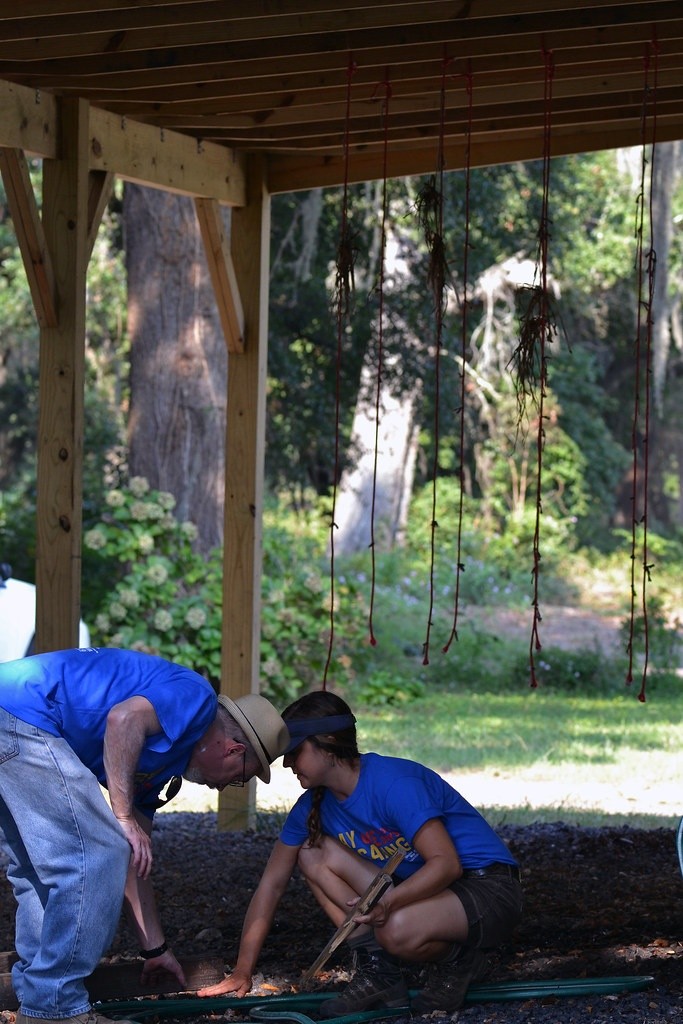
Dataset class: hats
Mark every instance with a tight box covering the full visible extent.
[217,693,292,784]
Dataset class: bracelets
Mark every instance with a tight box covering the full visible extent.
[140,940,170,959]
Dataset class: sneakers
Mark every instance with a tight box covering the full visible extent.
[320,953,411,1017]
[16,1006,132,1024]
[410,946,489,1012]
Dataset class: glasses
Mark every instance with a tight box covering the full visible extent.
[228,737,248,788]
[285,714,357,753]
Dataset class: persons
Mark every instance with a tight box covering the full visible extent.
[197,690,522,1014]
[0,648,290,1024]
